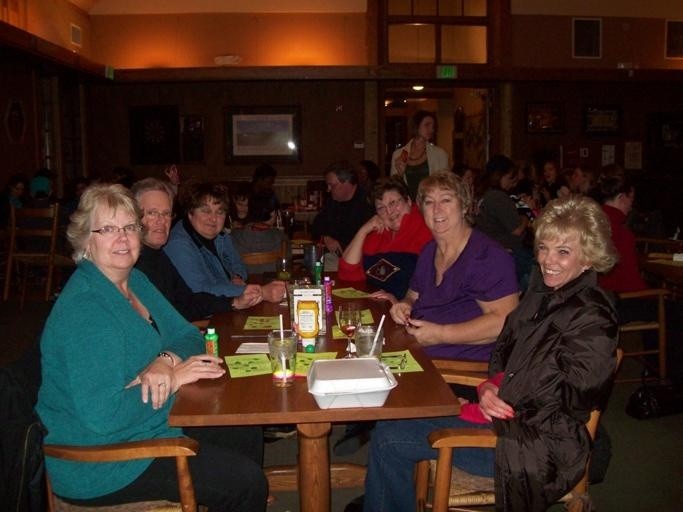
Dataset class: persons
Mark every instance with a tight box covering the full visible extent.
[165,132,220,222]
[338,178,431,290]
[249,165,279,222]
[333,168,521,459]
[132,177,264,323]
[454,163,473,216]
[310,164,374,257]
[591,173,672,416]
[391,109,450,204]
[346,196,620,512]
[570,161,596,195]
[474,154,529,236]
[29,170,53,204]
[1,175,25,217]
[162,184,287,302]
[35,183,270,511]
[535,154,571,215]
[224,183,254,230]
[233,191,286,285]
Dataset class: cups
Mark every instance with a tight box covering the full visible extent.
[354,325,383,360]
[339,303,361,358]
[274,256,293,281]
[267,330,297,386]
[303,244,325,276]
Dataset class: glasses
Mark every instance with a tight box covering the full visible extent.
[382,352,407,369]
[142,211,176,219]
[374,194,406,212]
[87,223,144,237]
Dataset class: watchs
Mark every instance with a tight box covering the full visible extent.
[156,352,174,366]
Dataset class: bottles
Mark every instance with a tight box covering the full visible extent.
[204,327,218,358]
[275,240,293,282]
[280,204,293,232]
[323,276,334,315]
[314,261,322,286]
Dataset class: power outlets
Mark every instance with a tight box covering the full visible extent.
[579,148,589,158]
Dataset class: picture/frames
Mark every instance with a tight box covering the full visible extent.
[644,113,683,163]
[223,107,303,164]
[525,101,566,133]
[623,140,643,170]
[582,105,624,135]
[601,146,615,166]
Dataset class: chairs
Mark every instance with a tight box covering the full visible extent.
[632,238,683,280]
[0,203,76,312]
[238,240,289,276]
[601,285,671,386]
[414,346,623,511]
[40,435,204,512]
[428,358,501,373]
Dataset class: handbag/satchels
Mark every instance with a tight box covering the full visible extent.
[362,252,419,301]
[229,223,290,277]
[625,367,683,419]
